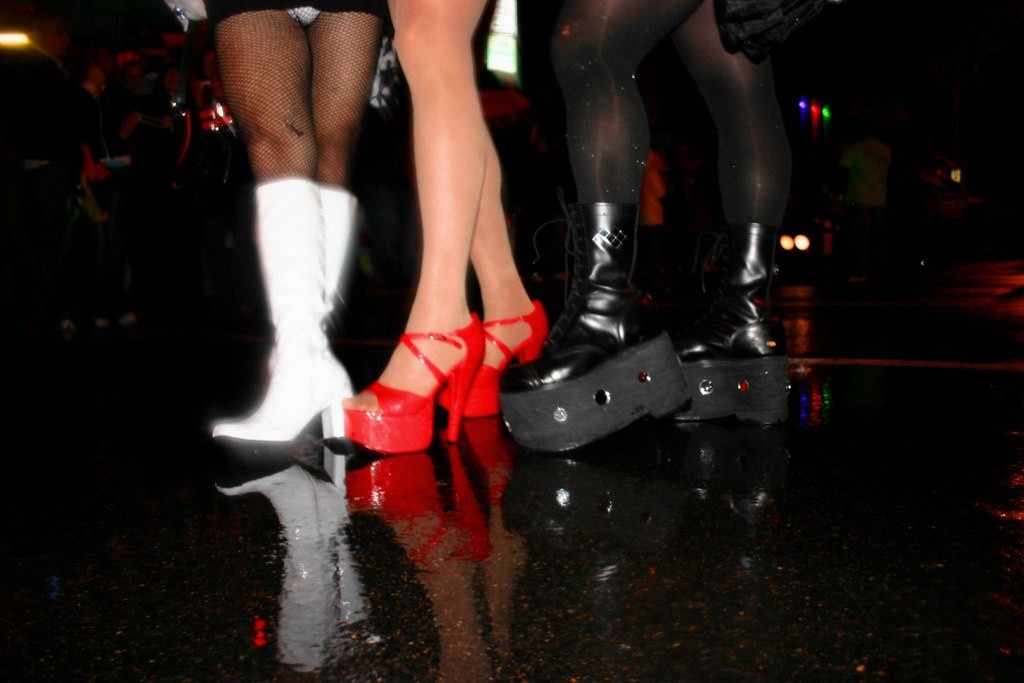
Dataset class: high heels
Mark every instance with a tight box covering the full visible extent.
[442,417,513,500]
[344,450,492,572]
[340,313,486,455]
[438,298,549,419]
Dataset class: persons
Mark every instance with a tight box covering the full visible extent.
[342,0,547,452]
[497,0,827,452]
[165,0,395,442]
[215,438,367,674]
[343,414,525,683]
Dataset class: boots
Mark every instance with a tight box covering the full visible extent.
[215,458,373,672]
[209,173,356,445]
[500,199,693,451]
[672,224,790,425]
